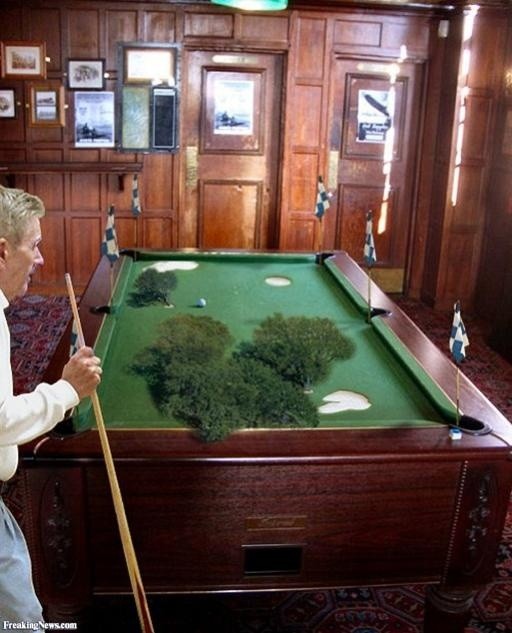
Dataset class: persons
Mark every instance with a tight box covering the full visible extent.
[0,186,104,633]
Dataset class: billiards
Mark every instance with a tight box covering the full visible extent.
[197,298,206,306]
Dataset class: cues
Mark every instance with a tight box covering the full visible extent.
[64,272,153,632]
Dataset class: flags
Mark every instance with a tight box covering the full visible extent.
[102,205,120,263]
[448,304,470,365]
[131,173,140,217]
[362,211,376,265]
[68,319,80,360]
[313,177,330,217]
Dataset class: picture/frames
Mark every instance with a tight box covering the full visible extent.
[0,39,107,130]
